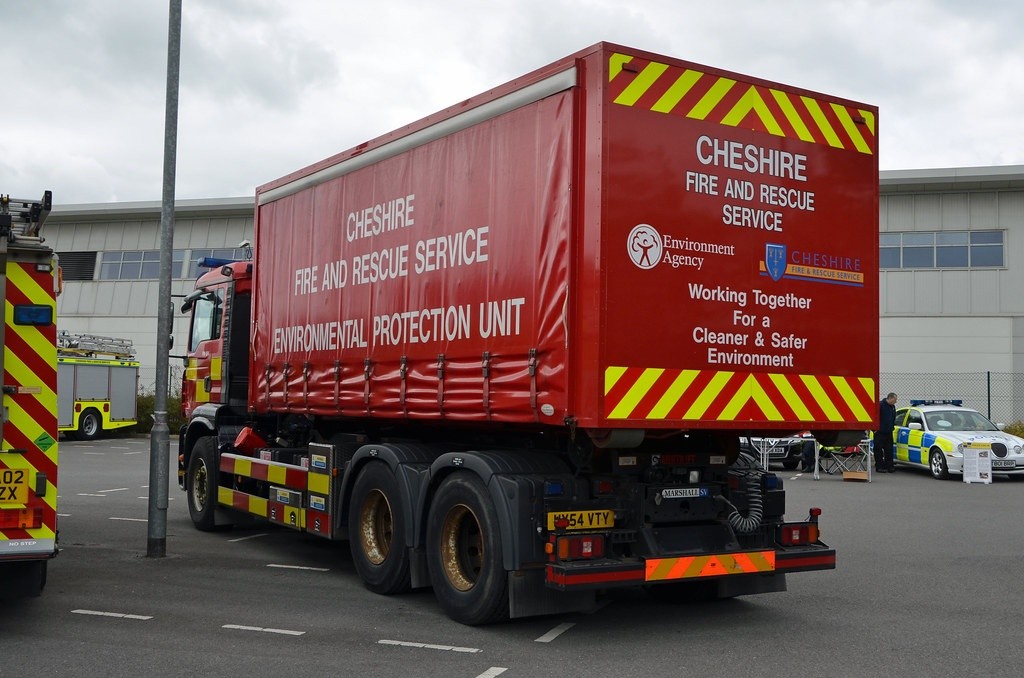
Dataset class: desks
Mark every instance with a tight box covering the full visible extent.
[744,438,817,483]
[816,440,872,483]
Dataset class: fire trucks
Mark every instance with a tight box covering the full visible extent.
[61,330,140,440]
[0,191,62,595]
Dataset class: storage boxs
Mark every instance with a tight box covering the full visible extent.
[843,471,868,481]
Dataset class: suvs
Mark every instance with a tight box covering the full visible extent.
[738,430,818,474]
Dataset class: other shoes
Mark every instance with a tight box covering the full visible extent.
[802,467,812,473]
[888,466,895,472]
[877,467,886,473]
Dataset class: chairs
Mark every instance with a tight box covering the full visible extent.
[944,414,961,426]
[931,416,942,425]
[818,449,868,474]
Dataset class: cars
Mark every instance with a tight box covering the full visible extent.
[871,398,1024,480]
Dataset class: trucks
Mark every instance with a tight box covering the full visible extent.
[170,40,882,623]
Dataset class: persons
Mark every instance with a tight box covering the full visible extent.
[801,442,843,473]
[871,393,897,473]
